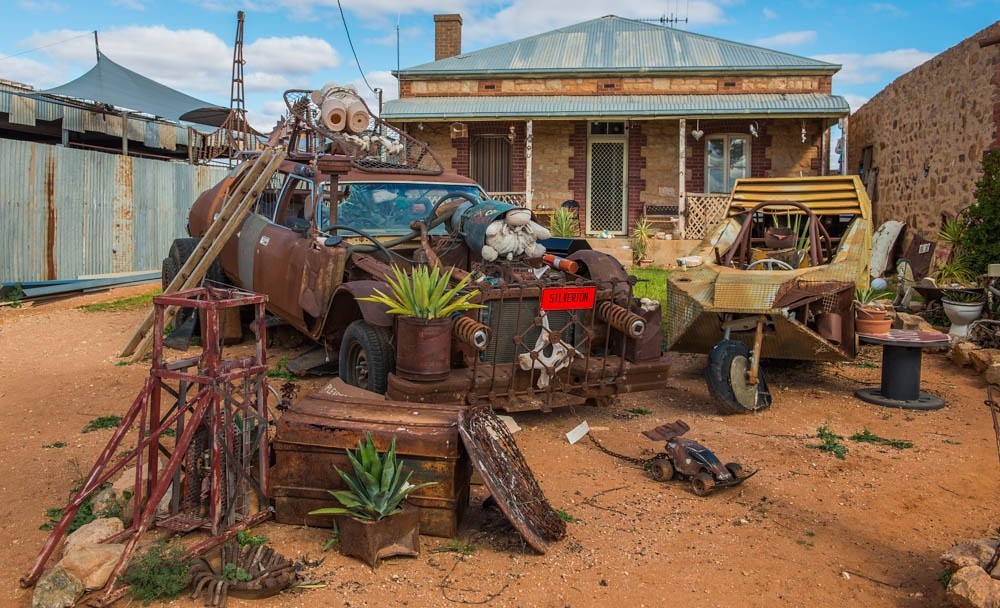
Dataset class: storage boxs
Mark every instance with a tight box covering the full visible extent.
[272,392,472,538]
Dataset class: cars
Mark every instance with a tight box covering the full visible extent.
[163,84,671,412]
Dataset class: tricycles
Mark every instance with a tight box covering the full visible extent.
[680,175,873,413]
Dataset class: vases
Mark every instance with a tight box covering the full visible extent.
[856,317,892,334]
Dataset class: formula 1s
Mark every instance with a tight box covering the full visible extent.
[641,420,759,495]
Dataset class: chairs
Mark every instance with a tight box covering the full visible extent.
[561,200,582,237]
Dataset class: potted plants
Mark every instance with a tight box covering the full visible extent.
[307,430,440,570]
[632,214,654,267]
[913,219,985,301]
[941,289,987,337]
[854,287,893,320]
[354,263,488,383]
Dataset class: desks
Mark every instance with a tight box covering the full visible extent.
[532,208,555,233]
